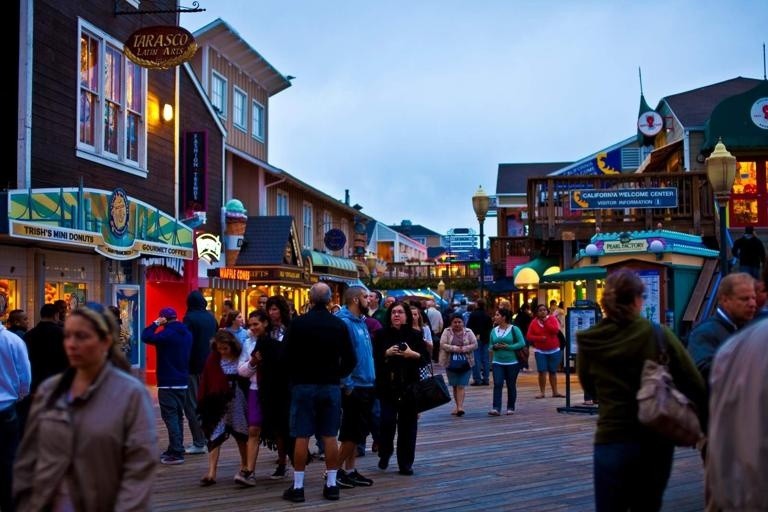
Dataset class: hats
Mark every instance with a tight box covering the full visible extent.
[158,306,177,318]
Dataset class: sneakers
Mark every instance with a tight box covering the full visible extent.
[281,484,305,504]
[399,463,414,476]
[378,453,393,469]
[233,466,258,487]
[270,466,289,479]
[321,483,340,501]
[185,445,207,454]
[160,448,187,466]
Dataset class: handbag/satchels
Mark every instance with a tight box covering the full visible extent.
[511,339,530,369]
[635,358,700,449]
[417,371,452,410]
[447,351,469,374]
[556,329,566,352]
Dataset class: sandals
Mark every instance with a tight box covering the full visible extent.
[199,475,218,486]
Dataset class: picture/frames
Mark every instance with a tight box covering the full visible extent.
[111,283,141,369]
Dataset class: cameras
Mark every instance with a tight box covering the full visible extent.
[396,342,408,352]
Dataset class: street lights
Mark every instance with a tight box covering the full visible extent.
[704,137,739,280]
[472,183,492,300]
[437,279,447,332]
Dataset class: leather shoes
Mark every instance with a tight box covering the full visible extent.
[321,465,375,489]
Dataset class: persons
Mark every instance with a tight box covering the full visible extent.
[0,292,158,511]
[142,283,564,504]
[688,225,768,512]
[575,267,705,512]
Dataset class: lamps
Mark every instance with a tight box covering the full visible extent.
[650,239,664,260]
[316,212,368,233]
[585,243,599,264]
[160,97,174,123]
